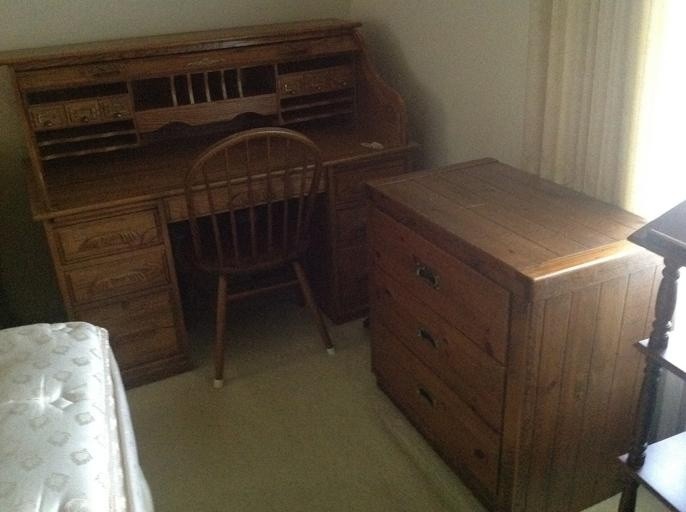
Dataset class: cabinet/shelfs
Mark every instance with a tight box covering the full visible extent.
[364,157,664,512]
[581,198,686,512]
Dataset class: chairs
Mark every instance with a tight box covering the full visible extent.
[172,127,339,389]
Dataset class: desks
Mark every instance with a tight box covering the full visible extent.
[0,19,420,391]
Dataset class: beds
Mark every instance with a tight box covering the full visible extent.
[0,321,156,511]
